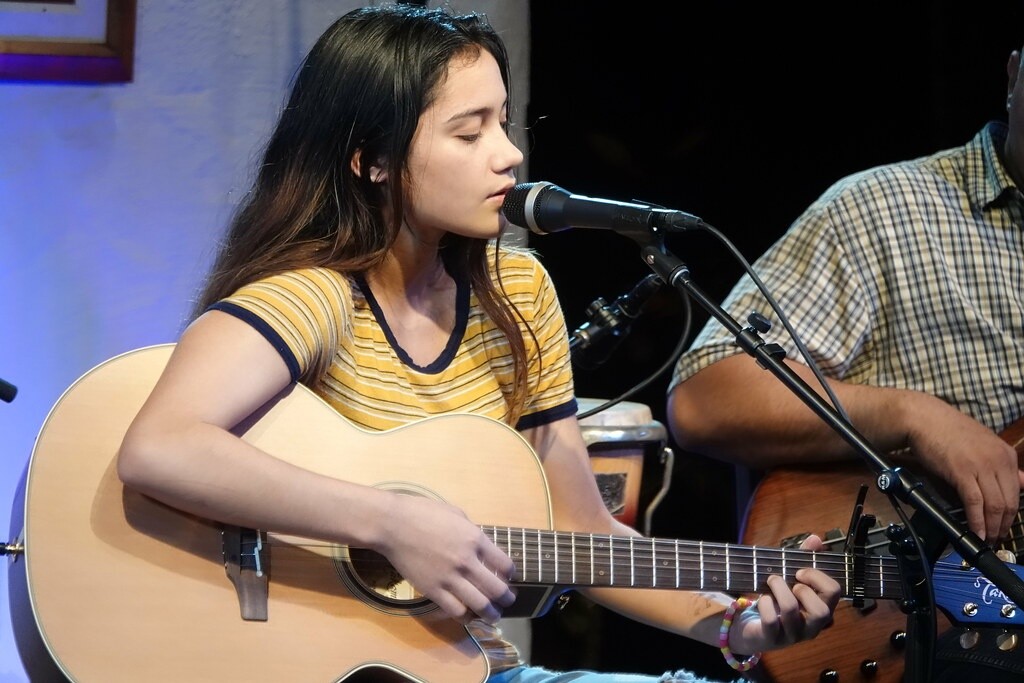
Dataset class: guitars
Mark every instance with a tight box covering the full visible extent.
[735,414,1024,683]
[6,341,1024,683]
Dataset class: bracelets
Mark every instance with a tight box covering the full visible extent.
[719,596,762,671]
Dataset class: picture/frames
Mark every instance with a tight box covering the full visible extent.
[0,0,139,86]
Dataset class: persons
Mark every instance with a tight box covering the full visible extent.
[115,6,838,682]
[669,39,1023,682]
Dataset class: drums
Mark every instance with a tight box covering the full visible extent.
[573,395,656,528]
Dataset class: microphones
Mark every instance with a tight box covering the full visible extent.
[502,181,701,235]
